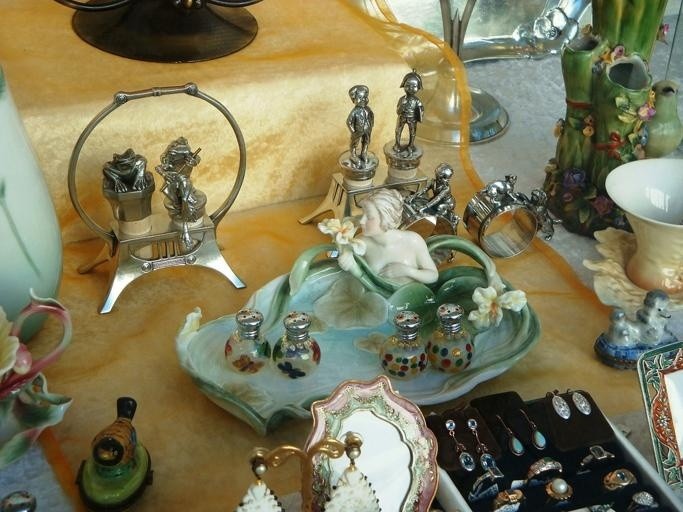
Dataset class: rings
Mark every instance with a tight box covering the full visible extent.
[464,443,670,511]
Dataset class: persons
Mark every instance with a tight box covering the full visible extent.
[345,84,375,166]
[404,162,457,217]
[392,68,424,152]
[338,187,440,288]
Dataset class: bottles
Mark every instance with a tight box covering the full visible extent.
[427,303,475,372]
[377,307,426,380]
[271,310,320,377]
[224,307,270,377]
